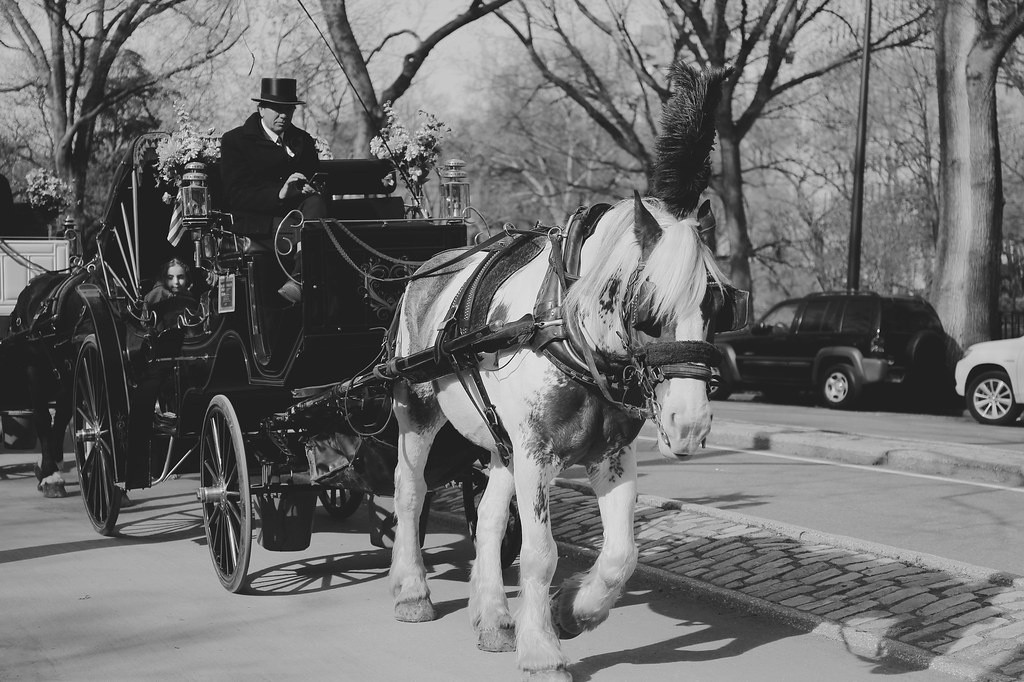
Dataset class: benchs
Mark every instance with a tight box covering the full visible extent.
[318,156,406,222]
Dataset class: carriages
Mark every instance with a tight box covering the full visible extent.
[1,65,743,682]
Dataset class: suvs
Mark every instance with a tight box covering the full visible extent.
[709,288,951,412]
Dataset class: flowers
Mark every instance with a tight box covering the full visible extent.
[368,99,452,197]
[155,98,223,190]
[18,167,81,218]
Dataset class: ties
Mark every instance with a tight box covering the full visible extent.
[276,136,287,153]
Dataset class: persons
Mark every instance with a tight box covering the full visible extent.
[141,256,199,335]
[219,78,333,305]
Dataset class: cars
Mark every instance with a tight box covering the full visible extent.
[954,334,1024,425]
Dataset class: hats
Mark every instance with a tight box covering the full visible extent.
[250,78,306,105]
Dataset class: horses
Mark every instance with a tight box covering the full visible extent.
[6,271,89,498]
[391,188,734,682]
